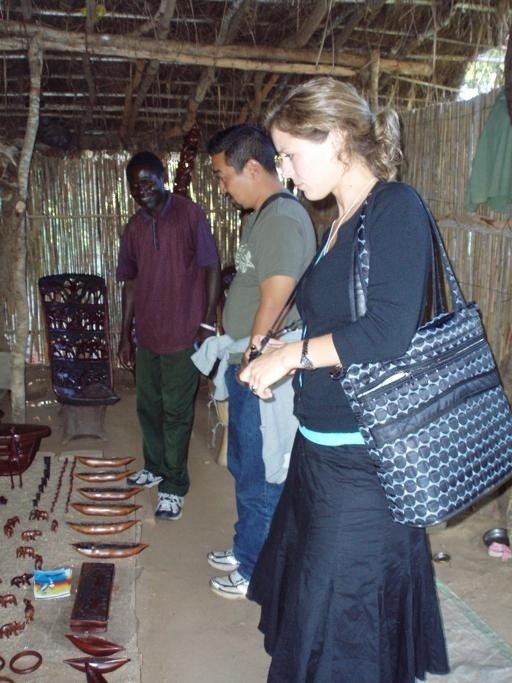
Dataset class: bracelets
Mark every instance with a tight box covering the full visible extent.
[199,322,216,332]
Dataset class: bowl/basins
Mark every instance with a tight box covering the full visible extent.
[483,525,510,555]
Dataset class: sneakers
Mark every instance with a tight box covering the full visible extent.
[154,491,184,520]
[127,468,164,488]
[206,549,241,571]
[208,570,249,599]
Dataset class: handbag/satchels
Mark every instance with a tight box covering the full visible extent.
[339,182,512,529]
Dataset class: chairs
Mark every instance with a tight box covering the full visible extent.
[38,273,120,445]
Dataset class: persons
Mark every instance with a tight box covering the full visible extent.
[238,78,451,682]
[205,124,317,600]
[114,152,222,523]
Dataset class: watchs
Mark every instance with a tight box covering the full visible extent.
[299,337,316,372]
